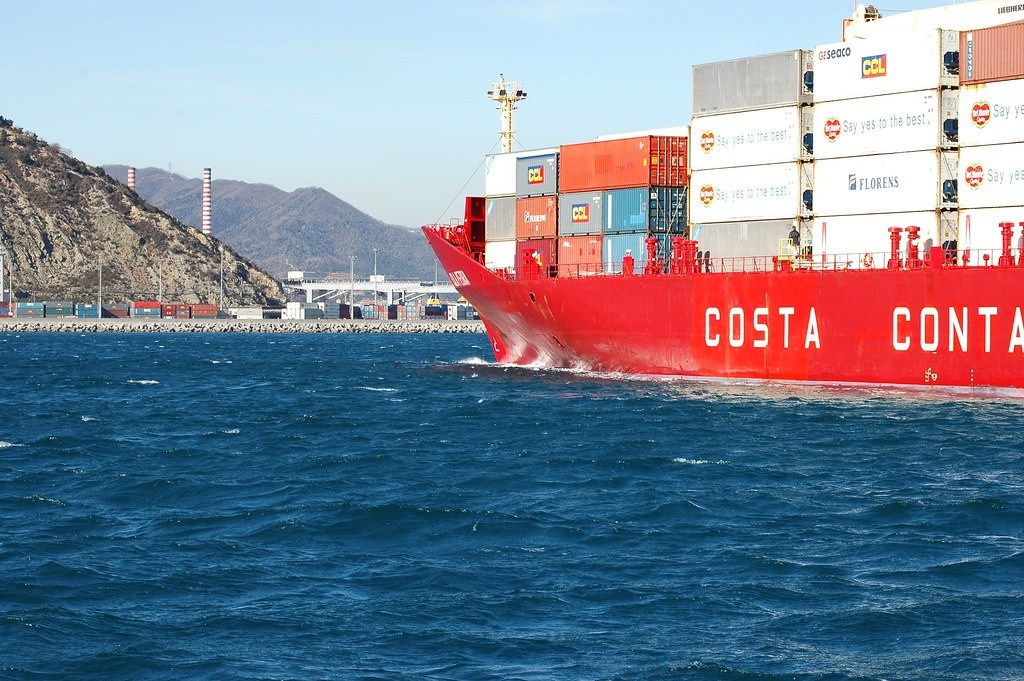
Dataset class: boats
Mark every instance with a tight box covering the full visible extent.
[418,0,1024,401]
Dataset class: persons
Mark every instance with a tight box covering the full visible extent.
[788,226,801,258]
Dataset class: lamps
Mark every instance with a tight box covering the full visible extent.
[515,90,523,97]
[523,92,527,97]
[499,89,506,95]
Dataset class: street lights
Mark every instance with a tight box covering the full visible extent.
[372,248,380,306]
[349,256,358,320]
